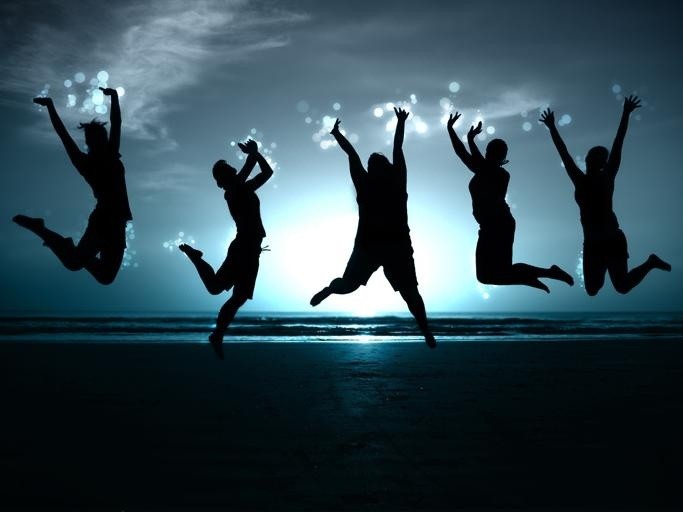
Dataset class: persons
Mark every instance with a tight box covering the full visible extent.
[537,93,672,296]
[447,110,575,294]
[308,104,437,350]
[177,138,274,363]
[10,84,132,287]
